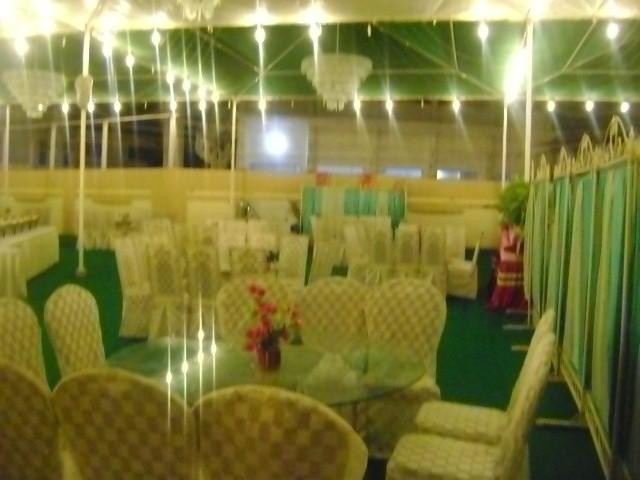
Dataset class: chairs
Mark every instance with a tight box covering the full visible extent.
[3,218,480,340]
[49,368,198,477]
[387,333,556,480]
[216,273,297,347]
[354,278,449,462]
[412,310,557,480]
[187,381,370,480]
[1,296,47,383]
[287,277,373,432]
[1,363,54,479]
[44,285,108,376]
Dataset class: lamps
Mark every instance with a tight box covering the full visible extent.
[2,36,66,118]
[301,22,372,113]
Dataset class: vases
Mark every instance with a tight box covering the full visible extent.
[256,340,281,368]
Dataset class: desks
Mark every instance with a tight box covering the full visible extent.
[485,223,528,315]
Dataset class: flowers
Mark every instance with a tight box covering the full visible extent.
[237,284,304,351]
